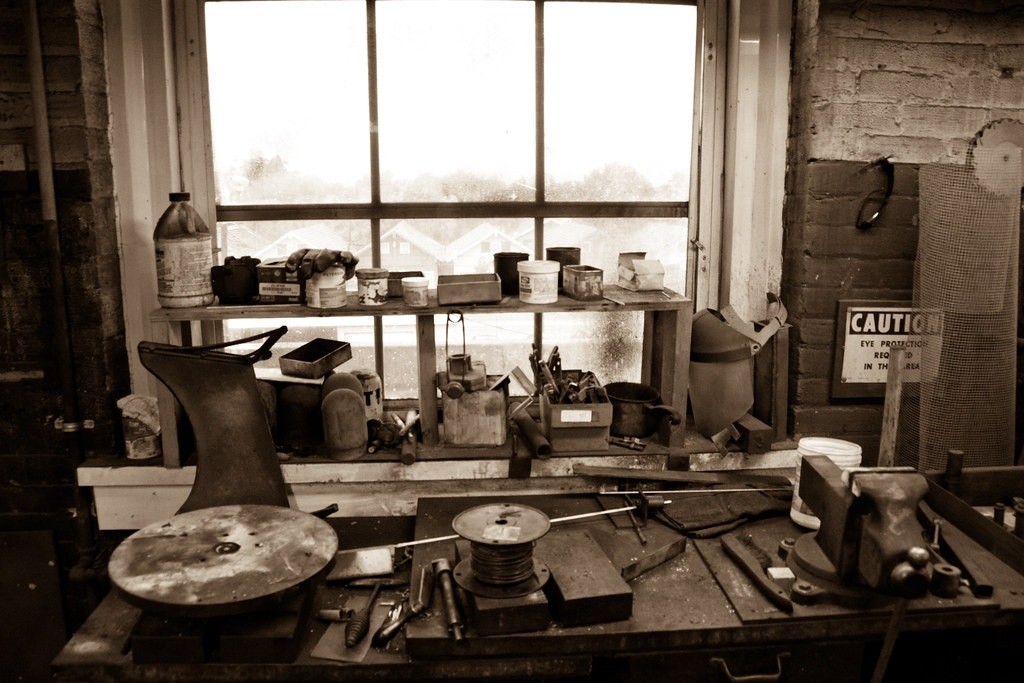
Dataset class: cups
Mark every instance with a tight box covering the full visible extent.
[546,247,581,289]
[401,277,429,307]
[494,253,529,294]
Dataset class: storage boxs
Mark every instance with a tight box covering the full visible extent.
[539,370,614,451]
[617,252,665,293]
[278,338,352,378]
[442,375,510,446]
[436,273,501,304]
[256,258,306,304]
[387,270,425,297]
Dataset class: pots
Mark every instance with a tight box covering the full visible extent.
[604,382,682,439]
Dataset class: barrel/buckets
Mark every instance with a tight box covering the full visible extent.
[790,437,861,529]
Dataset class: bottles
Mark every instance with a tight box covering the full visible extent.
[153,192,213,307]
